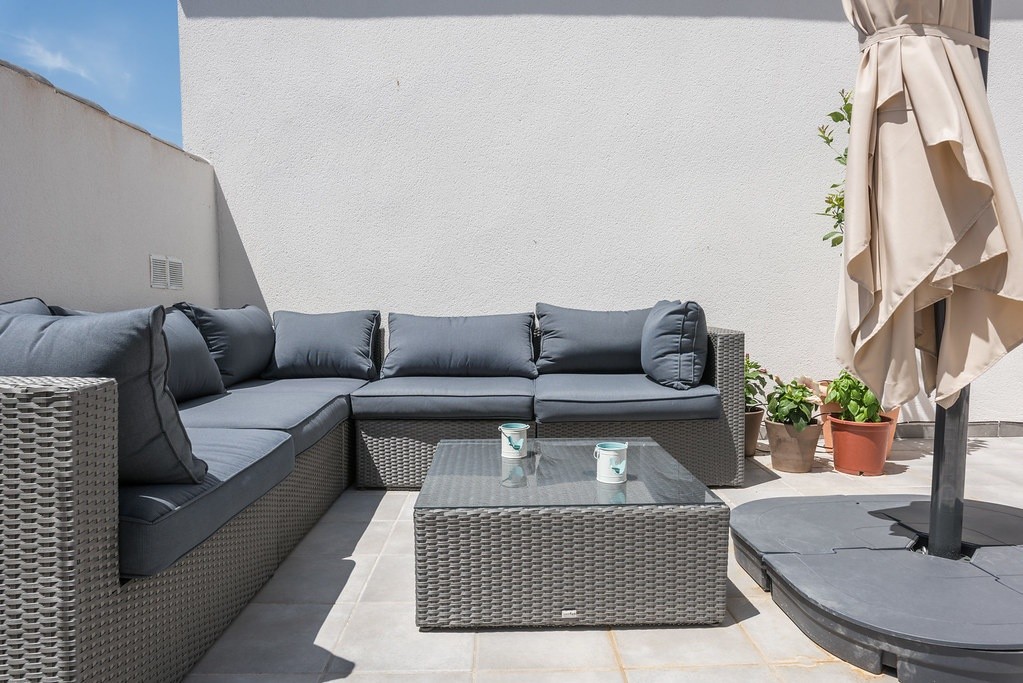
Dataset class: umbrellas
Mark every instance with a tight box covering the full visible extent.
[834,0,1023,561]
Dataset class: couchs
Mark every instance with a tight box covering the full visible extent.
[0,296,745,683]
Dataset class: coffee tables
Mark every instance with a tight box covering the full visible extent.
[413,437,730,629]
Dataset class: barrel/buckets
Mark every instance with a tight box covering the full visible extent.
[593,442,629,484]
[498,423,530,458]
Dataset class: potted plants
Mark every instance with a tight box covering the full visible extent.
[744,353,773,457]
[817,88,899,456]
[824,368,894,476]
[764,376,823,473]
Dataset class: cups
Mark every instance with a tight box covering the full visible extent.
[502,457,527,488]
[596,480,626,504]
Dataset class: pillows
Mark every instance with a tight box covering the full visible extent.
[379,312,538,380]
[641,299,708,390]
[0,297,52,315]
[535,302,653,373]
[260,310,381,378]
[52,306,226,404]
[186,302,275,388]
[0,305,210,485]
